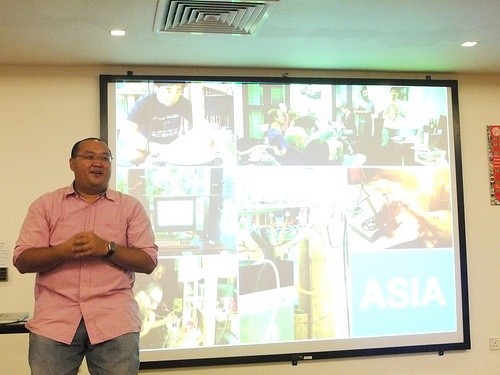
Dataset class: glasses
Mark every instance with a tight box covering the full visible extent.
[72,153,112,162]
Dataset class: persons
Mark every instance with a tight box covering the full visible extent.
[14,138,158,375]
[116,80,452,349]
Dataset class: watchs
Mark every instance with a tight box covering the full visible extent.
[105,240,117,258]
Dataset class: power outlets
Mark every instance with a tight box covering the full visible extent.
[489,336,500,351]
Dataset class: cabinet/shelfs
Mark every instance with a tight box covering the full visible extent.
[181,263,239,346]
